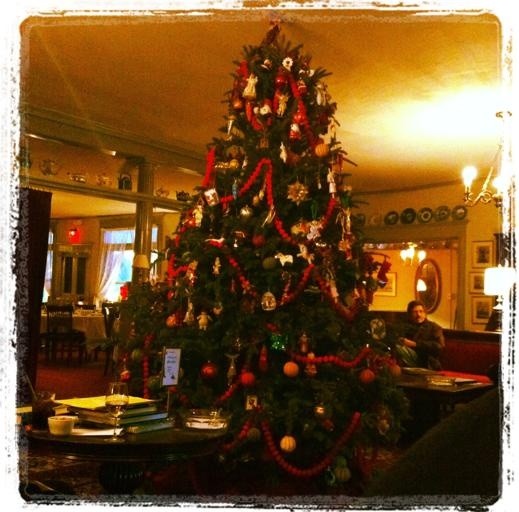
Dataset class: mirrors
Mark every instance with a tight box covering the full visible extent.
[352,220,466,330]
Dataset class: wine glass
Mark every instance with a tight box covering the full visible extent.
[104,382,129,445]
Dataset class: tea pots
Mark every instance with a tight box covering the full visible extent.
[39,157,191,202]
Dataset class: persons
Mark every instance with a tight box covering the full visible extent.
[389,300,446,367]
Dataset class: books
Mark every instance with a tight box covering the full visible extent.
[54,394,176,434]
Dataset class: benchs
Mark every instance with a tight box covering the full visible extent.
[408,327,501,402]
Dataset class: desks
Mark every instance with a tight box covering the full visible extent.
[15,395,239,496]
[385,362,491,444]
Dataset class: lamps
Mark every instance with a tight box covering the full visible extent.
[460,142,503,206]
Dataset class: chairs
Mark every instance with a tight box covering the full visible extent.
[37,302,124,374]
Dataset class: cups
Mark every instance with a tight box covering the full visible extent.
[48,415,79,437]
[73,309,102,317]
[32,390,55,434]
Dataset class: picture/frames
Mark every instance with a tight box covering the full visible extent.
[469,239,495,269]
[467,272,486,294]
[374,272,397,297]
[470,296,495,325]
[413,258,441,314]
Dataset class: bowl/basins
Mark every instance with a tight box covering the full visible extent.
[402,366,429,375]
[179,408,234,430]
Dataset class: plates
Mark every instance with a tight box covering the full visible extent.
[353,205,468,226]
[424,374,477,386]
